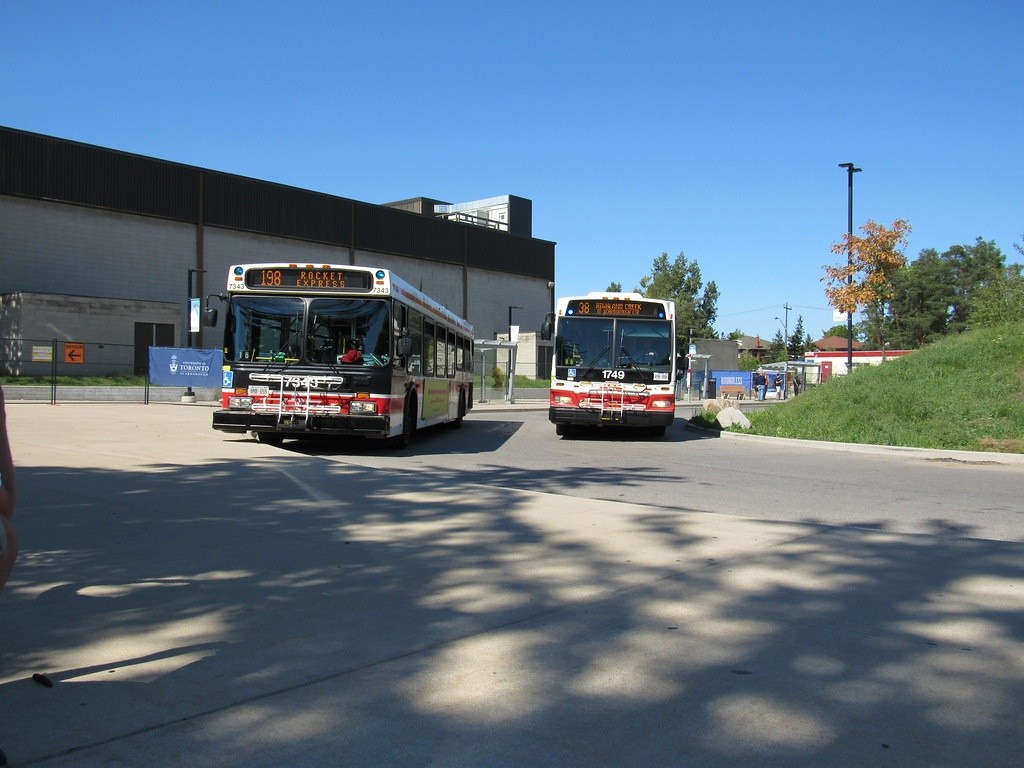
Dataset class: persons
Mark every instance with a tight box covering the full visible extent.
[762,372,769,399]
[775,374,782,400]
[793,375,800,395]
[755,371,766,400]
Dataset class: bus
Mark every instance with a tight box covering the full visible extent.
[203,261,476,451]
[540,291,689,440]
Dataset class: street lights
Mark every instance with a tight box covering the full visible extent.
[180,268,208,404]
[838,162,862,372]
[507,306,523,395]
[775,302,792,399]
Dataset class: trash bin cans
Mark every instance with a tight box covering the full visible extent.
[699,377,716,401]
